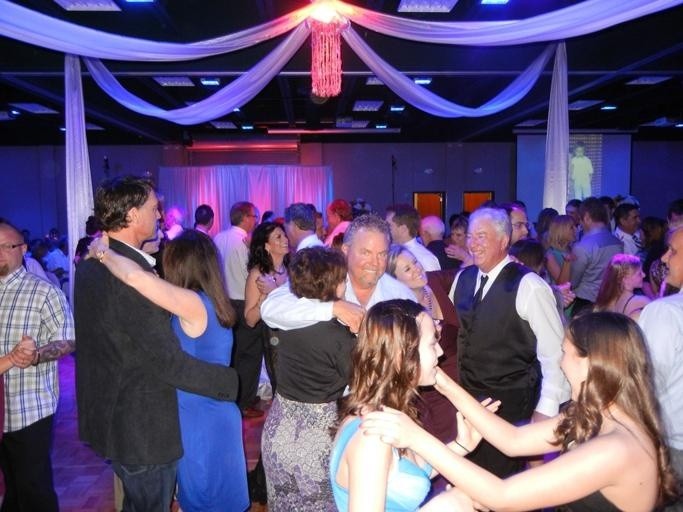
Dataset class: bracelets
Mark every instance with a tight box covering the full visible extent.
[453,439,471,454]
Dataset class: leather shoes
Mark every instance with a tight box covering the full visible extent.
[239,405,264,419]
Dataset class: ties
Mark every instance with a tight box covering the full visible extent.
[632,235,643,251]
[468,276,490,311]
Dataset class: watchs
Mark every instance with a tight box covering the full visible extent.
[31,348,42,366]
[94,246,110,262]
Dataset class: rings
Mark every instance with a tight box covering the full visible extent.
[271,276,277,283]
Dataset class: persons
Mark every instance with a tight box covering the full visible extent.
[74,164,238,512]
[312,211,327,241]
[260,209,276,223]
[666,198,683,228]
[613,203,642,257]
[71,217,101,262]
[281,203,317,253]
[602,195,617,218]
[260,247,357,512]
[543,216,579,286]
[163,207,185,240]
[374,311,676,512]
[447,215,474,265]
[141,225,164,255]
[187,203,215,235]
[44,227,61,241]
[384,205,440,271]
[385,242,444,318]
[32,242,69,271]
[536,206,557,227]
[498,201,576,306]
[239,220,290,327]
[629,216,665,274]
[443,208,570,421]
[258,214,418,338]
[2,334,36,369]
[82,225,249,512]
[207,201,264,418]
[417,216,462,270]
[329,298,505,512]
[0,220,78,512]
[584,254,651,319]
[635,224,683,473]
[324,199,354,245]
[563,198,581,225]
[569,142,593,201]
[567,196,623,314]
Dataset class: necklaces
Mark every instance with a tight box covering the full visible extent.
[421,289,433,316]
[271,267,287,275]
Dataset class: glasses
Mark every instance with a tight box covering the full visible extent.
[0,241,24,255]
[508,223,533,231]
[247,214,259,219]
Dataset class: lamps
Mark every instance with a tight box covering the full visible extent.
[309,1,336,23]
